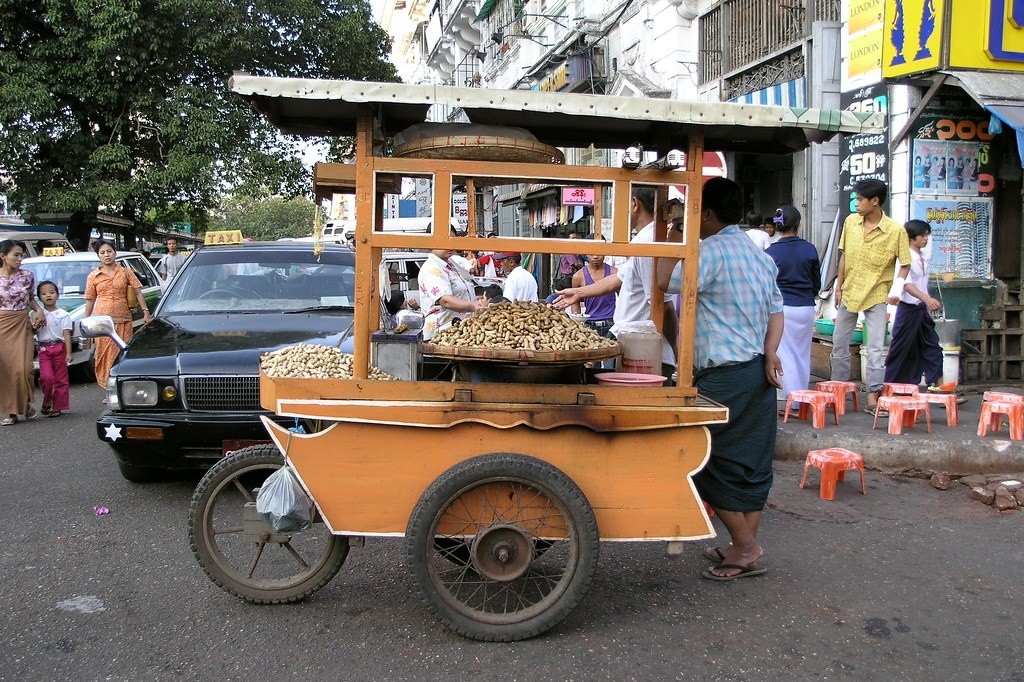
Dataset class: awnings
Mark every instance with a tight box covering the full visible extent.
[928,71,1024,172]
[494,184,579,208]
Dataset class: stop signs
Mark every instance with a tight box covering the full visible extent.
[673,151,729,201]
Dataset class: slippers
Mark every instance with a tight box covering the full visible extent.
[779,410,798,419]
[863,404,889,417]
[1,417,19,425]
[702,558,768,580]
[26,405,37,420]
[704,546,725,562]
[939,398,968,407]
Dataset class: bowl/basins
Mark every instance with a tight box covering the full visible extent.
[813,318,835,335]
[850,329,862,343]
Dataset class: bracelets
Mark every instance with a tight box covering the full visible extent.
[414,306,420,311]
[888,277,906,301]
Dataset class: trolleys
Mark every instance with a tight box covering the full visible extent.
[186,77,886,644]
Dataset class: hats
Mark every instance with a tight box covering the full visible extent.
[385,290,404,315]
[492,250,520,259]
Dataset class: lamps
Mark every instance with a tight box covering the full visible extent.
[490,13,569,45]
[475,33,547,63]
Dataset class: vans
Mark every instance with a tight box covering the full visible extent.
[149,244,196,280]
[0,231,76,269]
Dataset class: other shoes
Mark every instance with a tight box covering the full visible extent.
[41,396,51,417]
[49,409,61,416]
[102,398,107,403]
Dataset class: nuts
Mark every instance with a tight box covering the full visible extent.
[426,299,619,350]
[260,344,401,381]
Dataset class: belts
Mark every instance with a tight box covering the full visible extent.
[585,320,614,327]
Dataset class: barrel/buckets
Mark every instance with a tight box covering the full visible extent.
[859,345,888,384]
[933,317,959,348]
[594,372,668,387]
[861,318,890,346]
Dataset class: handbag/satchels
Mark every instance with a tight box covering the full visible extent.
[256,464,316,534]
[124,267,138,308]
[553,254,580,291]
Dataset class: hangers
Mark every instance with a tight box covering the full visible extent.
[533,193,557,202]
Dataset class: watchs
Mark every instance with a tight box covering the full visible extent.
[143,308,149,311]
[671,223,683,232]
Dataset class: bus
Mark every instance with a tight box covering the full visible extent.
[321,217,462,241]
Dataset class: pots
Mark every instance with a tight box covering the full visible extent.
[452,360,587,384]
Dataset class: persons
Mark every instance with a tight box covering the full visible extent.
[84,239,151,404]
[382,290,422,329]
[553,182,655,324]
[657,176,784,581]
[0,240,47,427]
[129,247,151,263]
[36,239,70,257]
[572,233,621,370]
[831,179,912,418]
[883,219,968,409]
[744,201,783,251]
[159,237,188,296]
[764,205,821,420]
[417,222,512,341]
[492,251,539,305]
[553,230,585,314]
[28,281,73,418]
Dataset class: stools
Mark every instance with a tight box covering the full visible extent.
[872,396,932,436]
[977,391,1024,441]
[881,382,919,415]
[815,380,859,415]
[798,447,867,501]
[913,393,959,428]
[782,390,839,429]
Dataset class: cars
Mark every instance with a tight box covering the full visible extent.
[18,245,195,390]
[79,230,391,484]
[276,235,430,318]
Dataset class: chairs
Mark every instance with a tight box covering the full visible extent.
[221,275,269,299]
[67,273,87,293]
[296,273,347,300]
